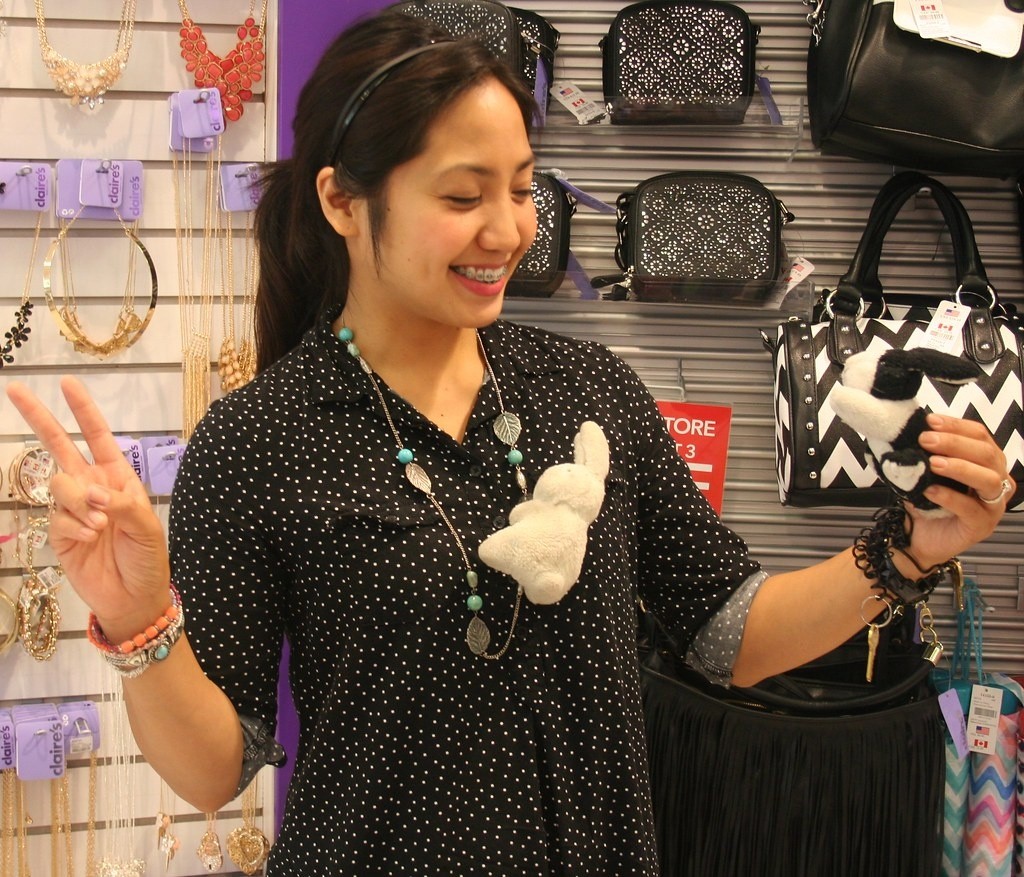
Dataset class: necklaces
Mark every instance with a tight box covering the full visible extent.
[0,213,42,372]
[32,0,137,111]
[177,0,272,123]
[168,129,263,446]
[336,322,535,661]
[2,660,270,877]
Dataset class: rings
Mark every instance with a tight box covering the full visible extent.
[979,478,1011,505]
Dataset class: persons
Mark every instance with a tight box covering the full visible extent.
[4,11,1012,876]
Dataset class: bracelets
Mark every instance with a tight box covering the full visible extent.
[85,583,185,679]
[854,507,947,606]
[15,582,60,662]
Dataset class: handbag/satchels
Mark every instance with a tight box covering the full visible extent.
[803,0,1024,178]
[759,166,1024,511]
[597,0,782,126]
[589,170,796,305]
[629,599,946,877]
[505,172,618,301]
[386,0,561,129]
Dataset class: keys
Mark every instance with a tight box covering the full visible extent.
[858,595,893,684]
[945,558,965,613]
